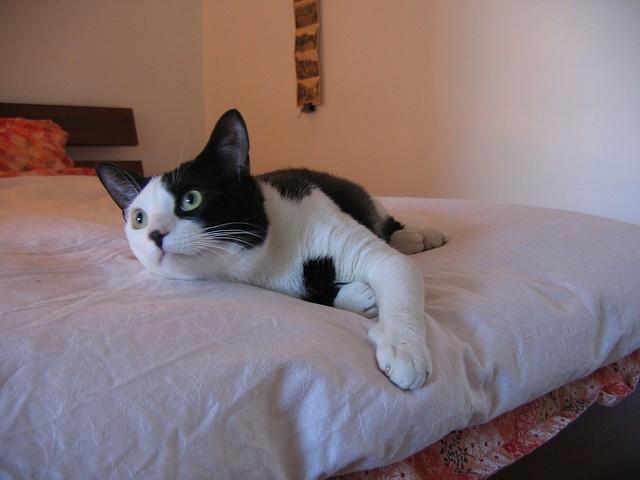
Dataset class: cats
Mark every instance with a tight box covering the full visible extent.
[95,109,448,390]
